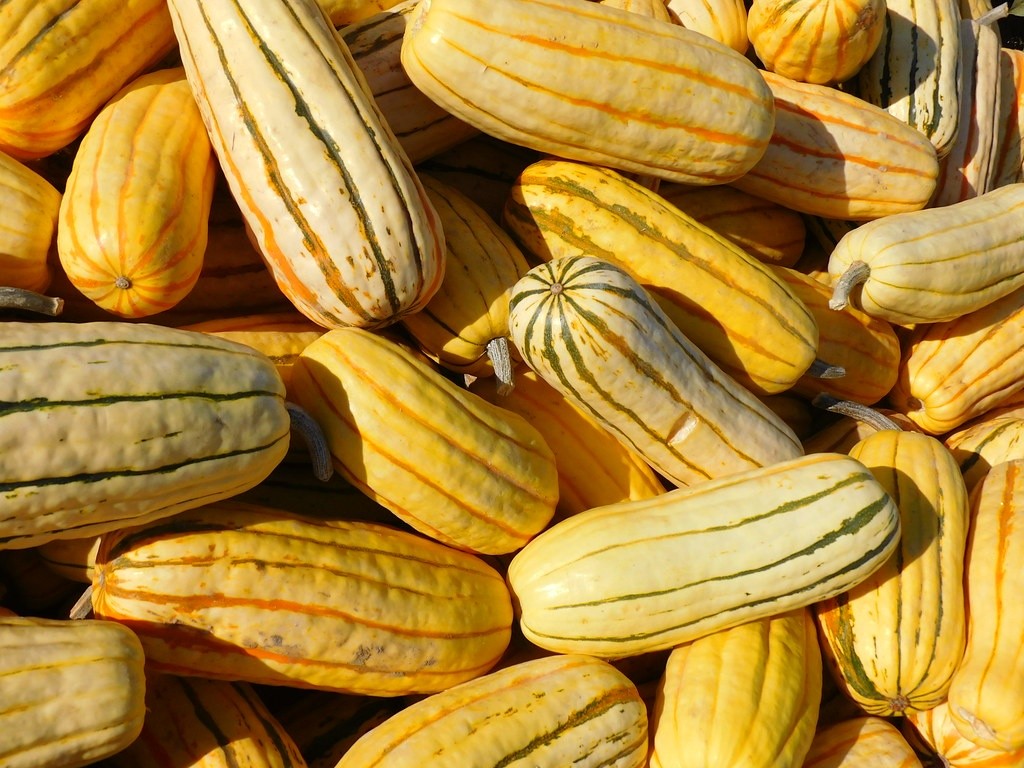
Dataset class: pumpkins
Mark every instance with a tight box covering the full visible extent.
[0,0,1024,768]
